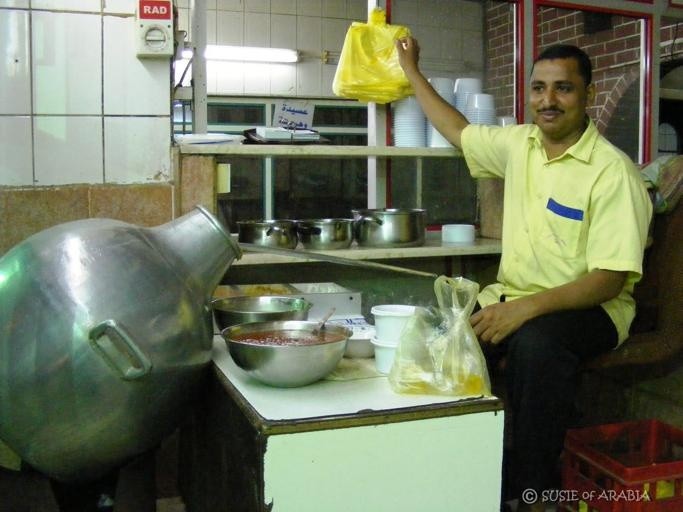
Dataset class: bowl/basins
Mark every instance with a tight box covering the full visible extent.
[210,292,314,337]
[389,75,511,151]
[221,319,353,389]
[440,224,476,244]
[340,327,374,358]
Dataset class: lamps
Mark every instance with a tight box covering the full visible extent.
[181,44,475,72]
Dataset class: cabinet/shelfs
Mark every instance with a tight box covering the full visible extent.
[206,102,288,200]
[287,104,366,201]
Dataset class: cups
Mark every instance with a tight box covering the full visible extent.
[369,306,423,374]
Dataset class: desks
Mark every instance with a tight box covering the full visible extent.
[200,317,504,512]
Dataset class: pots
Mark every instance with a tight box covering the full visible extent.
[233,217,300,250]
[351,206,427,248]
[295,217,354,250]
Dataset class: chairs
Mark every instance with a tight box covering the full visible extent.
[562,198,683,468]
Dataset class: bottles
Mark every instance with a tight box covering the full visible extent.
[0,204,243,483]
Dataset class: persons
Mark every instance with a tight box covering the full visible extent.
[395,35,655,500]
